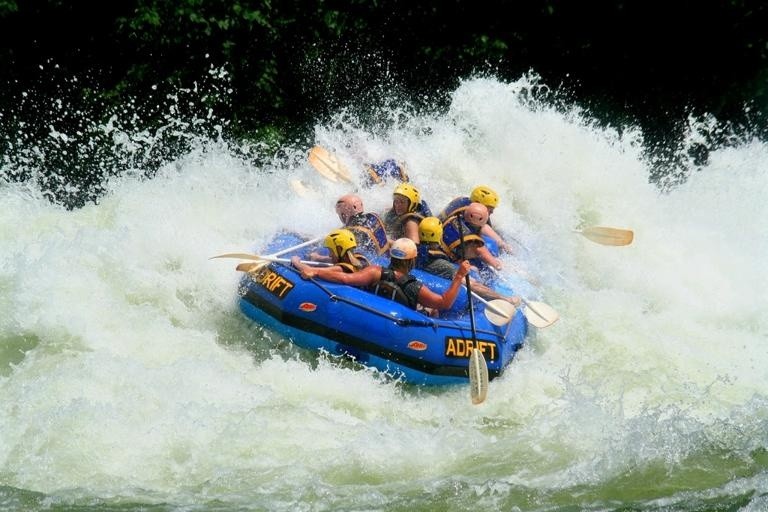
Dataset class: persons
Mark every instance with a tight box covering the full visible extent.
[385,182,425,247]
[307,194,389,262]
[289,229,370,290]
[299,237,471,312]
[439,202,512,274]
[438,186,499,228]
[354,155,410,190]
[415,215,520,307]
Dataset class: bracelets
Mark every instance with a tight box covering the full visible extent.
[315,268,320,279]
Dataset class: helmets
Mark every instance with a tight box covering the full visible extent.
[335,194,365,224]
[322,228,359,260]
[463,202,491,227]
[417,216,445,246]
[393,182,422,214]
[389,237,419,260]
[470,185,500,208]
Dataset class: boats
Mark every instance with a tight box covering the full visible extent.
[239,232,526,387]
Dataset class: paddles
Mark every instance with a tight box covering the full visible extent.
[237,237,325,273]
[576,226,634,246]
[306,148,359,188]
[208,252,338,267]
[484,264,560,328]
[456,214,489,406]
[460,283,517,326]
